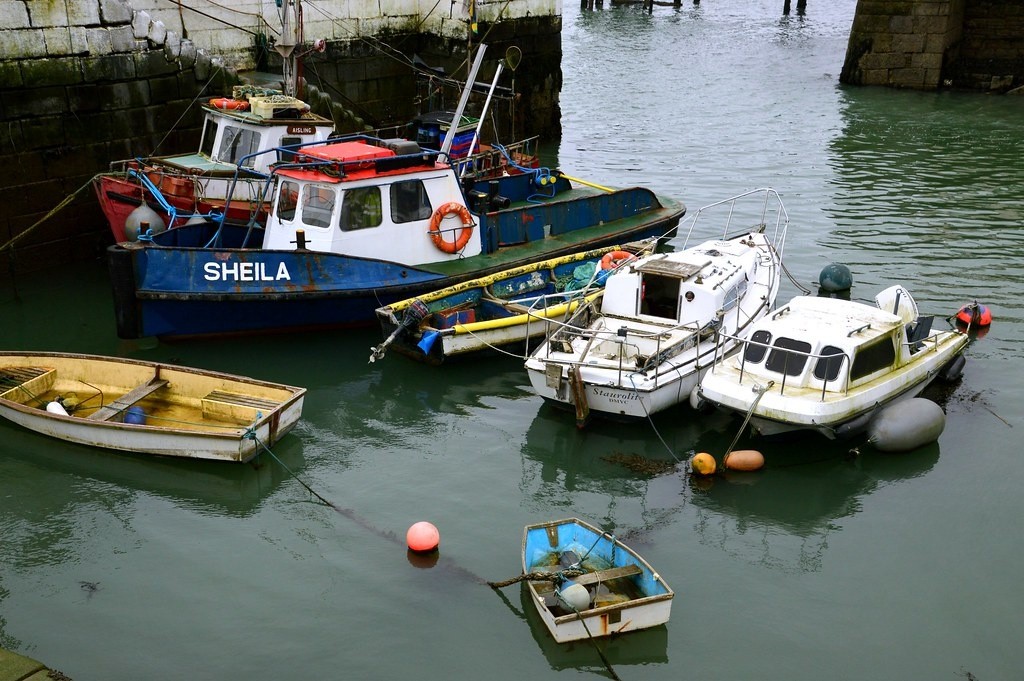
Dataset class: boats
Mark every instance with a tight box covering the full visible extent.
[521,517,675,644]
[519,570,670,672]
[87,1,997,451]
[0,350,310,464]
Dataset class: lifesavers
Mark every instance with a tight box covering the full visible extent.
[430,204,474,254]
[599,251,638,273]
[210,97,250,110]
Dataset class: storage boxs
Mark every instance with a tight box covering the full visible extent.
[417,116,481,160]
[145,167,194,197]
[232,89,306,119]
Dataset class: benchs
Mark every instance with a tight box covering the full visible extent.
[569,564,641,586]
[482,297,537,313]
[86,379,169,421]
